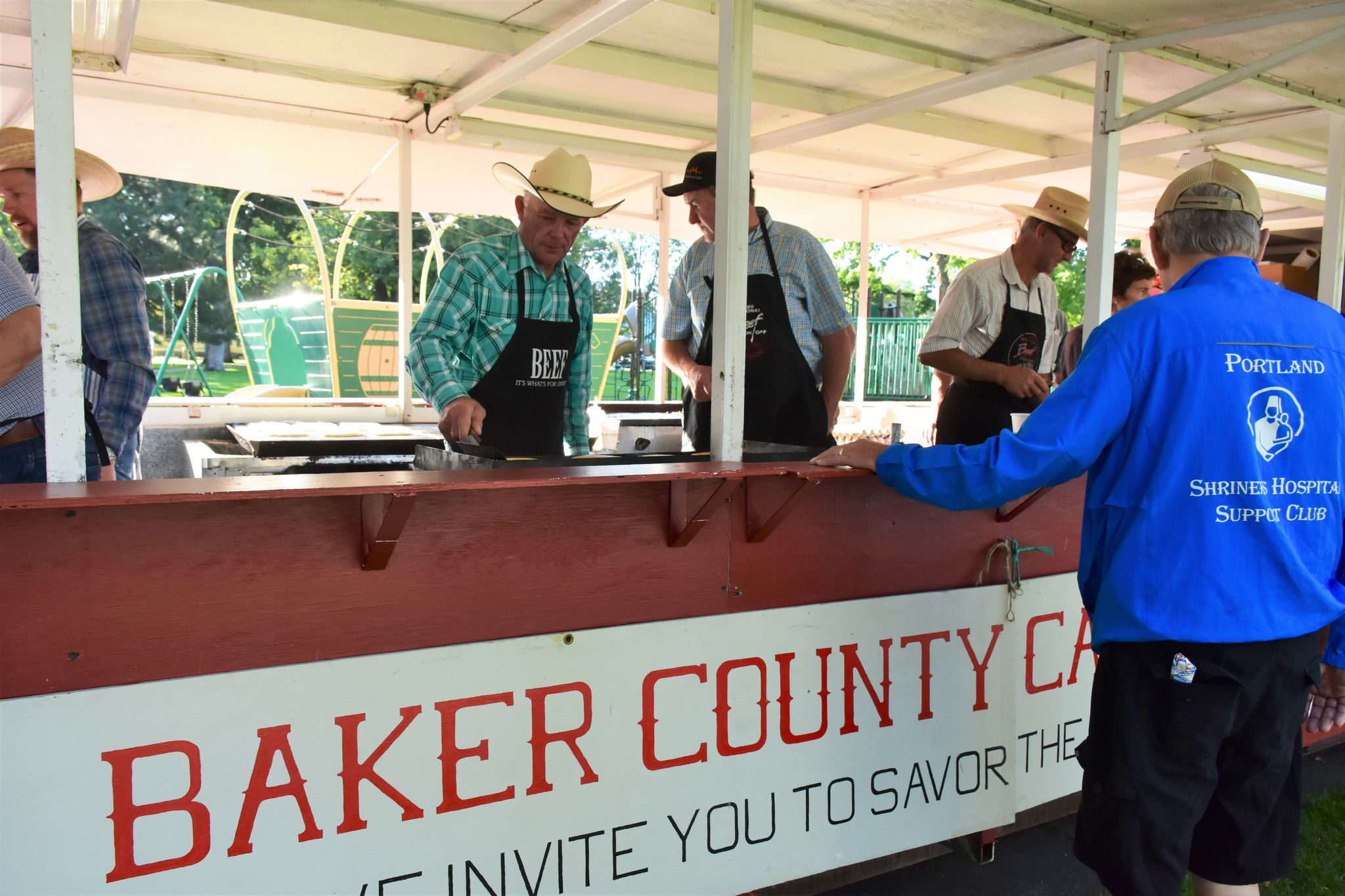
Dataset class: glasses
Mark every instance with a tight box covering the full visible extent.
[1047,222,1077,254]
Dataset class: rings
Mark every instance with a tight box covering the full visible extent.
[839,447,845,456]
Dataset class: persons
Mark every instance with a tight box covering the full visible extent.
[662,152,855,451]
[1044,307,1072,395]
[0,239,102,484]
[918,184,1087,445]
[1058,248,1157,386]
[407,147,625,459]
[810,159,1345,896]
[0,127,158,483]
[921,369,953,447]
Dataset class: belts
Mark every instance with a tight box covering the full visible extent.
[0,415,45,448]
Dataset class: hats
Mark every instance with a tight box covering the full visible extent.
[0,127,123,202]
[1002,186,1090,243]
[1154,158,1264,226]
[662,151,755,197]
[492,147,626,218]
